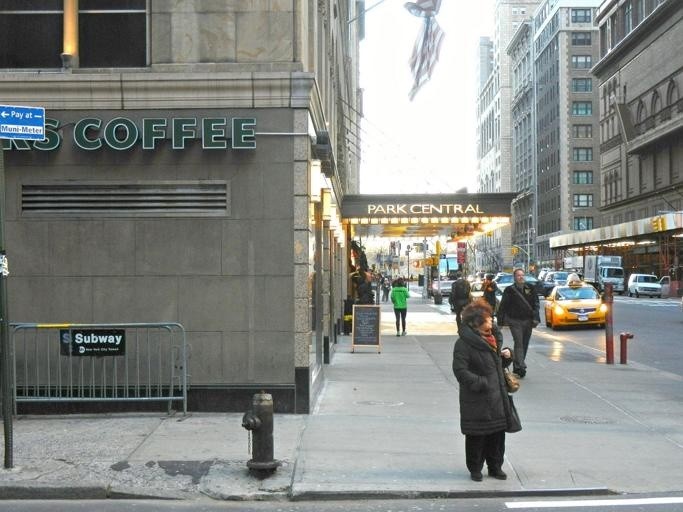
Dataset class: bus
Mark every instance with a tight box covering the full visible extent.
[425,253,463,297]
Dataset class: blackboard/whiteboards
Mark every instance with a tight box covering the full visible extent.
[351,304,382,348]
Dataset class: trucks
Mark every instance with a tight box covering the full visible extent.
[560,255,626,296]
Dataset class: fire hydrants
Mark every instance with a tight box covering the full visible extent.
[236,385,284,474]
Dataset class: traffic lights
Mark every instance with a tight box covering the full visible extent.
[660,217,666,232]
[510,246,515,255]
[515,247,519,254]
[652,217,659,233]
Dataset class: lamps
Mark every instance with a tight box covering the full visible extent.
[58,51,73,71]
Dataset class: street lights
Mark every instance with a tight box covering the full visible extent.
[406,245,412,292]
[526,214,536,273]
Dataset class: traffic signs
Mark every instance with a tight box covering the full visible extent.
[0,104,44,139]
[430,254,446,259]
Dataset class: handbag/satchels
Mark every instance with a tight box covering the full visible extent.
[533,308,541,329]
[502,359,521,393]
[503,383,522,433]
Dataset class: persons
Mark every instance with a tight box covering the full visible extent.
[451,299,515,482]
[355,271,392,305]
[479,274,498,324]
[389,278,410,336]
[451,270,471,334]
[496,268,540,378]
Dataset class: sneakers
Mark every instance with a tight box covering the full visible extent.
[396,330,400,336]
[513,367,526,378]
[471,471,483,481]
[488,467,507,480]
[402,331,407,336]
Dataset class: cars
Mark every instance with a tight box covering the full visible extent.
[401,259,672,314]
[542,270,608,330]
